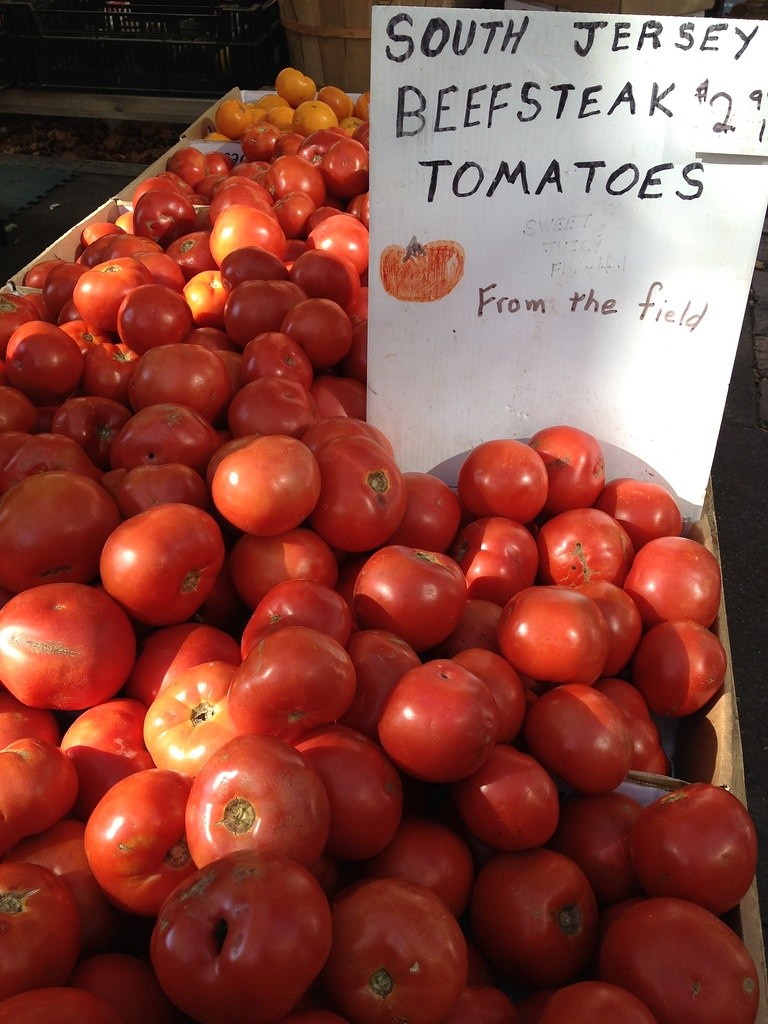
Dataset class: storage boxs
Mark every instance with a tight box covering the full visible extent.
[0,2,767,1023]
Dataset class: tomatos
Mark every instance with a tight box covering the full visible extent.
[0,68,759,1024]
